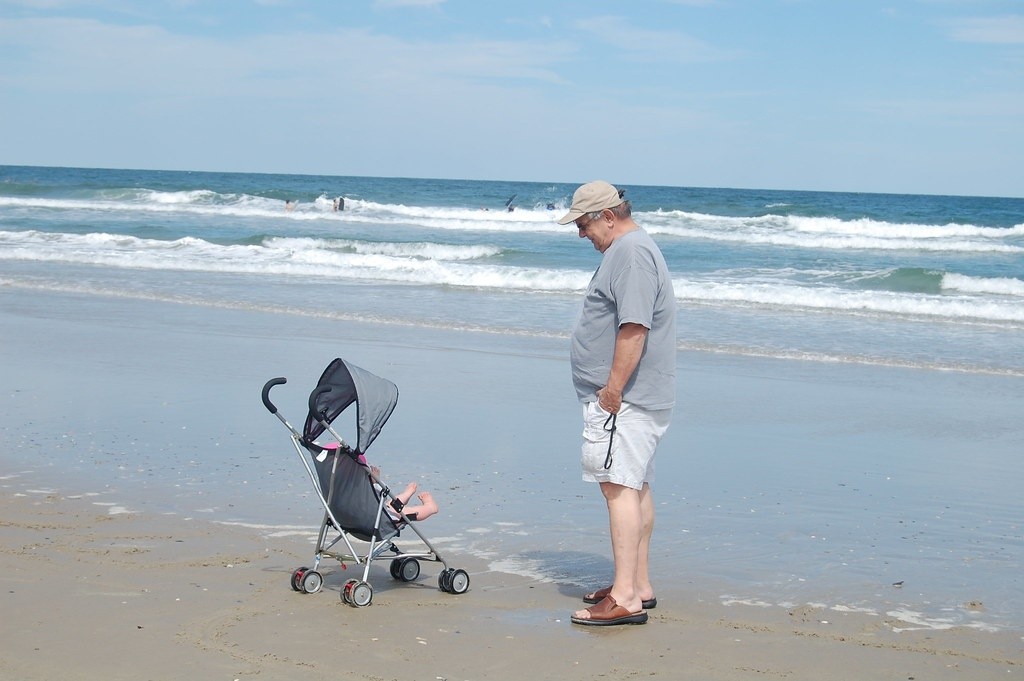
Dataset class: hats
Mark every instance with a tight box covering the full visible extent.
[557,181,624,226]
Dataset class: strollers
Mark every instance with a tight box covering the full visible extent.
[261,357,471,609]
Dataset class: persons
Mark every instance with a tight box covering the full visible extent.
[333,196,344,211]
[323,442,438,521]
[546,200,556,210]
[557,180,676,625]
[480,205,489,212]
[509,204,515,212]
[285,199,295,211]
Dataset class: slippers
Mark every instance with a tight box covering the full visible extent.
[570,593,648,625]
[583,584,657,609]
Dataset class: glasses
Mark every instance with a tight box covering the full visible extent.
[577,214,598,230]
